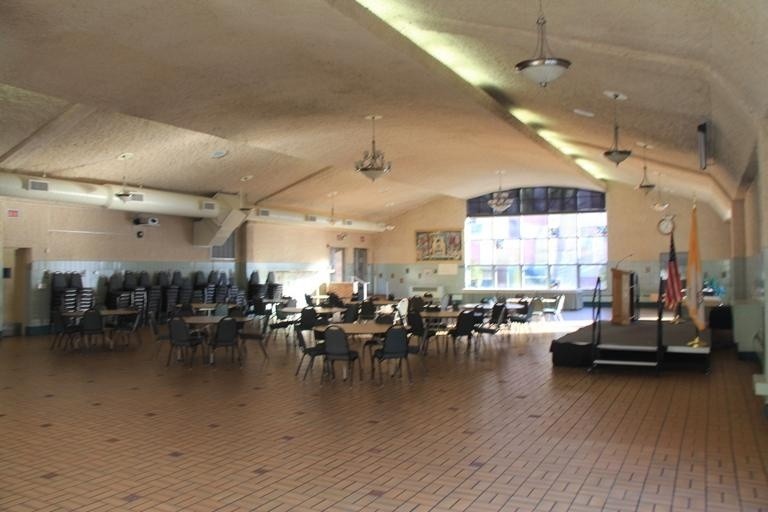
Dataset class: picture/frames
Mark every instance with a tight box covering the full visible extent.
[416,230,462,261]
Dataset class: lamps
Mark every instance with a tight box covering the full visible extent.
[514,0,572,88]
[354,116,391,182]
[603,94,631,166]
[636,146,655,196]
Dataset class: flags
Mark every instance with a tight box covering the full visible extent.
[664,234,682,312]
[680,205,706,331]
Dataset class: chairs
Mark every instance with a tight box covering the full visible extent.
[51,270,566,385]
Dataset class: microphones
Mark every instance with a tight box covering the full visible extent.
[615,254,633,268]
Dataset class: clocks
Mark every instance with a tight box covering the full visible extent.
[657,219,675,235]
